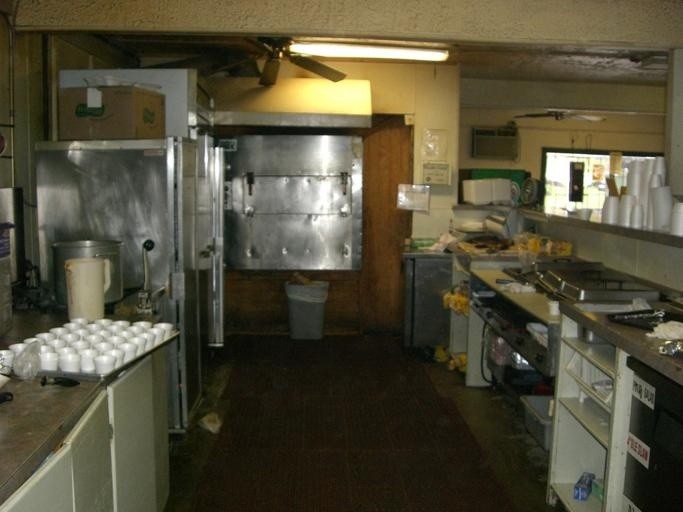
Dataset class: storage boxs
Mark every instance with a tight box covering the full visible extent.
[463,178,513,206]
[54,66,200,140]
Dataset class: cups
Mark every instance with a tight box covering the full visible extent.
[63,257,111,328]
[577,207,592,223]
[602,156,683,237]
[0,317,174,376]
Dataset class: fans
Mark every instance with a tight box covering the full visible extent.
[514,107,609,126]
[211,36,347,87]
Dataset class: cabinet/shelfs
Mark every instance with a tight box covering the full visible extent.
[547,299,683,512]
[1,354,160,510]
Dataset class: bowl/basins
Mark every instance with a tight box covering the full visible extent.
[576,324,608,345]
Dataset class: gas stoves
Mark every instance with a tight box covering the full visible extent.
[503,255,661,306]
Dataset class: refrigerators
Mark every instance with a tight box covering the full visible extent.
[32,135,202,431]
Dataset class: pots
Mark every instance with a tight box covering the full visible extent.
[51,239,122,306]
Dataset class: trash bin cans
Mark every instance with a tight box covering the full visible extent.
[286,281,328,339]
